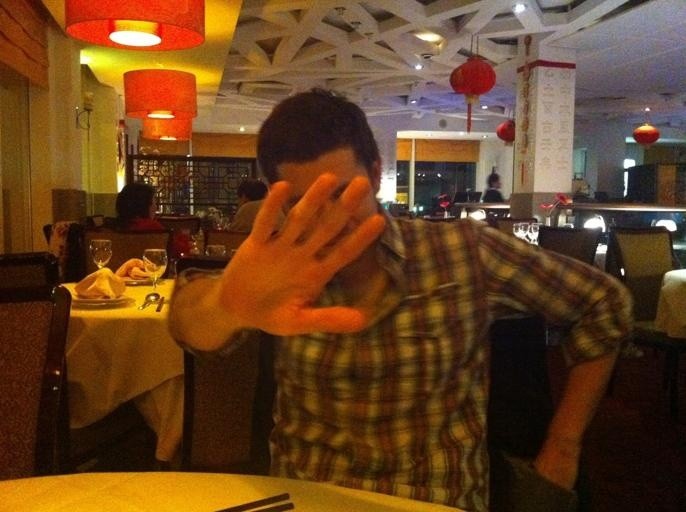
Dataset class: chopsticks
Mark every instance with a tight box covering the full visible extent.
[214,492,295,512]
[156,296,165,312]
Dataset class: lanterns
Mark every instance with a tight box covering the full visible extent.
[496,120,515,146]
[449,56,496,134]
[632,122,659,150]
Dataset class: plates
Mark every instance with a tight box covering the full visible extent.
[120,276,159,286]
[71,294,130,308]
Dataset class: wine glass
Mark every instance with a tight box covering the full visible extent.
[89,239,113,269]
[512,222,543,245]
[143,248,169,293]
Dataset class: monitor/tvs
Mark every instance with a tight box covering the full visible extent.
[454,191,482,203]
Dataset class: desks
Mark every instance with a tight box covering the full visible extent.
[1,470,493,512]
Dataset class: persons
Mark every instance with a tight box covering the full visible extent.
[115,182,156,219]
[482,173,503,202]
[231,180,269,233]
[169,88,635,512]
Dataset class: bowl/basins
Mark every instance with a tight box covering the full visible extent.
[205,244,226,257]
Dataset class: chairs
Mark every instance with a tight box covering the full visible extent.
[1,287,70,478]
[3,218,681,422]
[474,310,553,510]
[172,256,279,474]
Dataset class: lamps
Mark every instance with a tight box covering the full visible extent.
[62,0,206,141]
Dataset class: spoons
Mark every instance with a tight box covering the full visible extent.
[138,293,160,310]
